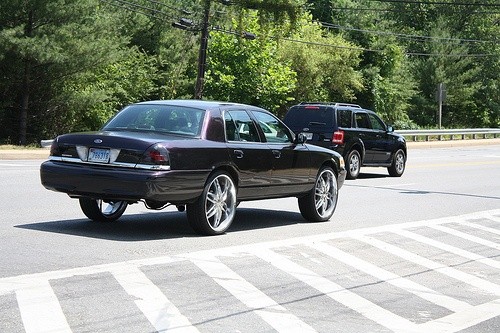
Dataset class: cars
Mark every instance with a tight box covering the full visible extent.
[40,99,345,234]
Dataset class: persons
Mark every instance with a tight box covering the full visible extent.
[226,121,246,142]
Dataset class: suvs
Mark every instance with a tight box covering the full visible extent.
[286,101,408,179]
